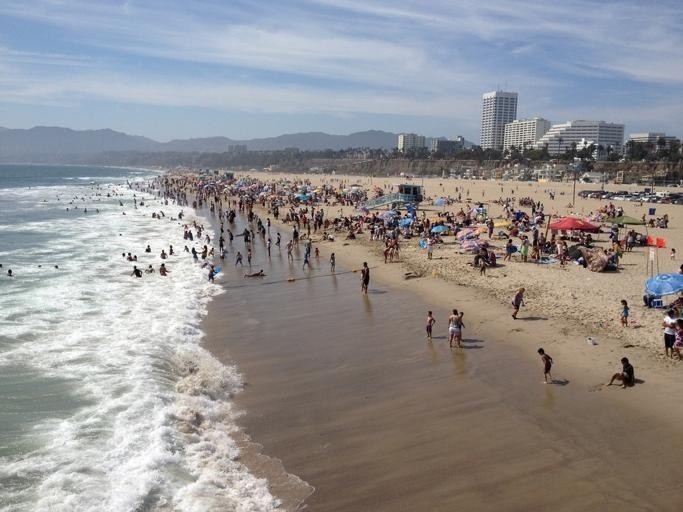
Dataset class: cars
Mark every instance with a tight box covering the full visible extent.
[578,189,683,206]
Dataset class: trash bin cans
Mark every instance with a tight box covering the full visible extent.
[648,208,656,215]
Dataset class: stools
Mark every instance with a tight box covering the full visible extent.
[651,298,663,307]
